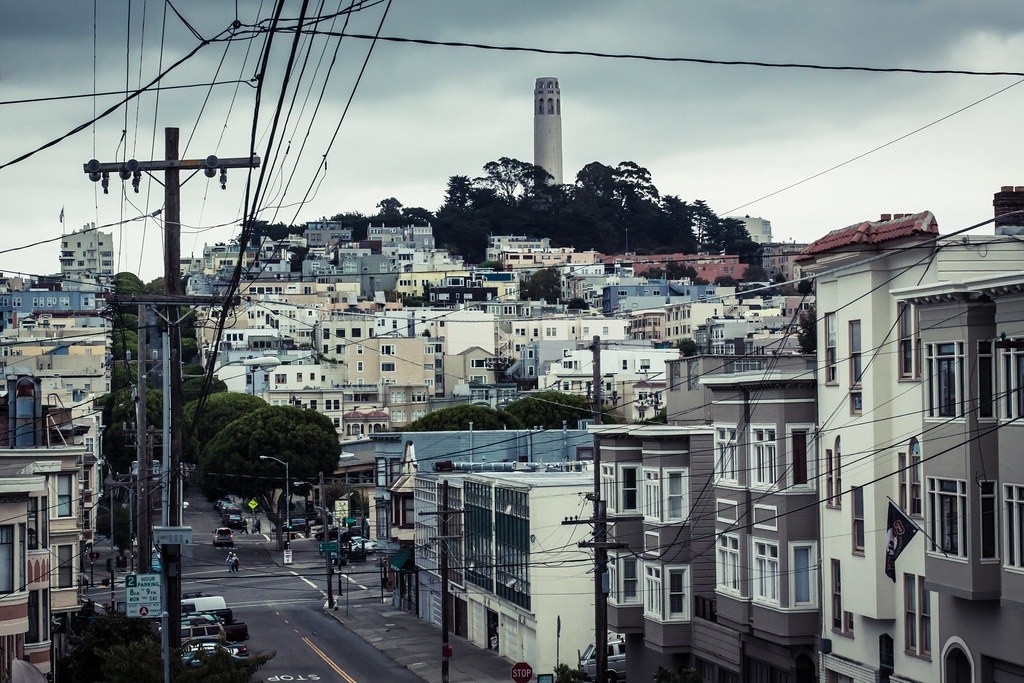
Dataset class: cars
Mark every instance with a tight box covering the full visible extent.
[315,528,338,541]
[212,527,234,547]
[282,518,308,533]
[339,530,378,553]
[215,497,243,528]
[159,593,251,680]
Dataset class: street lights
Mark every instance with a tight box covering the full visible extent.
[294,473,333,610]
[260,456,292,564]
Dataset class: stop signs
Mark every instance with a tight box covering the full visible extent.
[512,662,532,683]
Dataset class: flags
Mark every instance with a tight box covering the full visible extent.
[59,208,64,223]
[885,502,919,584]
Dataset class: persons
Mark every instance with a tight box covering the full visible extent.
[254,516,262,534]
[226,551,240,574]
[239,517,249,534]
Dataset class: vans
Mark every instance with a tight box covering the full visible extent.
[578,640,625,683]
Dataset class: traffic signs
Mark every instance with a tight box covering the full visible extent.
[125,575,160,619]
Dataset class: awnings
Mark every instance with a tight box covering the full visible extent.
[390,547,415,572]
